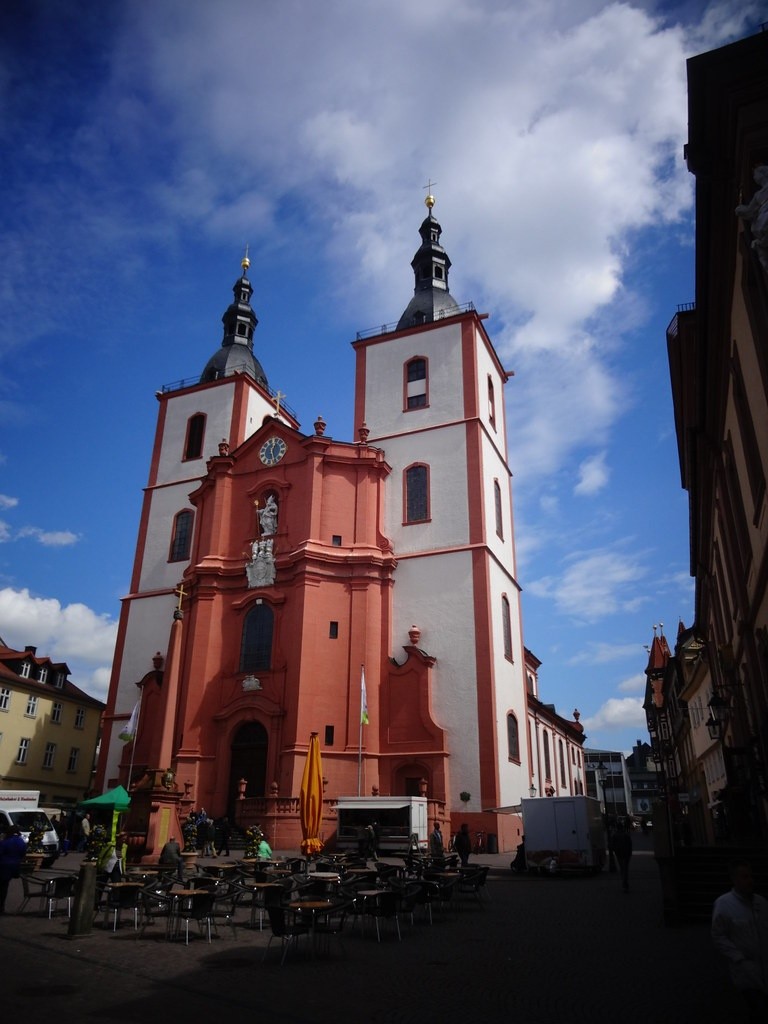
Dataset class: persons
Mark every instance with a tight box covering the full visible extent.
[256,494,277,532]
[602,812,649,832]
[453,824,471,867]
[364,822,380,862]
[711,860,768,1024]
[182,809,232,858]
[610,823,632,889]
[430,823,443,857]
[258,837,272,867]
[0,825,26,914]
[51,810,104,856]
[158,836,180,864]
[91,831,138,910]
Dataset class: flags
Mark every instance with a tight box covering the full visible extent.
[118,696,141,741]
[361,671,369,724]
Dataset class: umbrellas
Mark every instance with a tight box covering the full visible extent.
[298,731,325,855]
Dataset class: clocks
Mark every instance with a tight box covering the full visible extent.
[258,438,286,465]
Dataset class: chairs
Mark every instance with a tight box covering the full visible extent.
[19,856,488,967]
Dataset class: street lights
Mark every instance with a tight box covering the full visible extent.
[596,759,607,817]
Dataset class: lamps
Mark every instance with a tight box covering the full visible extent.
[529,784,536,797]
[706,685,732,739]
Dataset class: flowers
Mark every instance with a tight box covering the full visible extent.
[24,821,46,851]
[247,826,262,856]
[183,820,201,849]
[84,827,107,857]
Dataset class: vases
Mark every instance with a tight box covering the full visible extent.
[26,854,43,870]
[183,852,196,868]
[68,863,95,936]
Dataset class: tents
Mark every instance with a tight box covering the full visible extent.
[78,785,132,812]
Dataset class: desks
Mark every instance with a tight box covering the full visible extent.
[130,870,159,881]
[243,859,284,869]
[210,864,233,876]
[310,872,340,895]
[289,902,333,949]
[249,883,278,931]
[171,890,208,939]
[266,870,292,879]
[108,882,144,931]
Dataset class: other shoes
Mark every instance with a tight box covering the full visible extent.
[212,856,218,859]
[224,854,229,856]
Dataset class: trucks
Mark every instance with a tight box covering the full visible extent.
[482,796,607,878]
[0,788,63,869]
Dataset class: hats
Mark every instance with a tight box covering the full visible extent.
[365,825,373,830]
